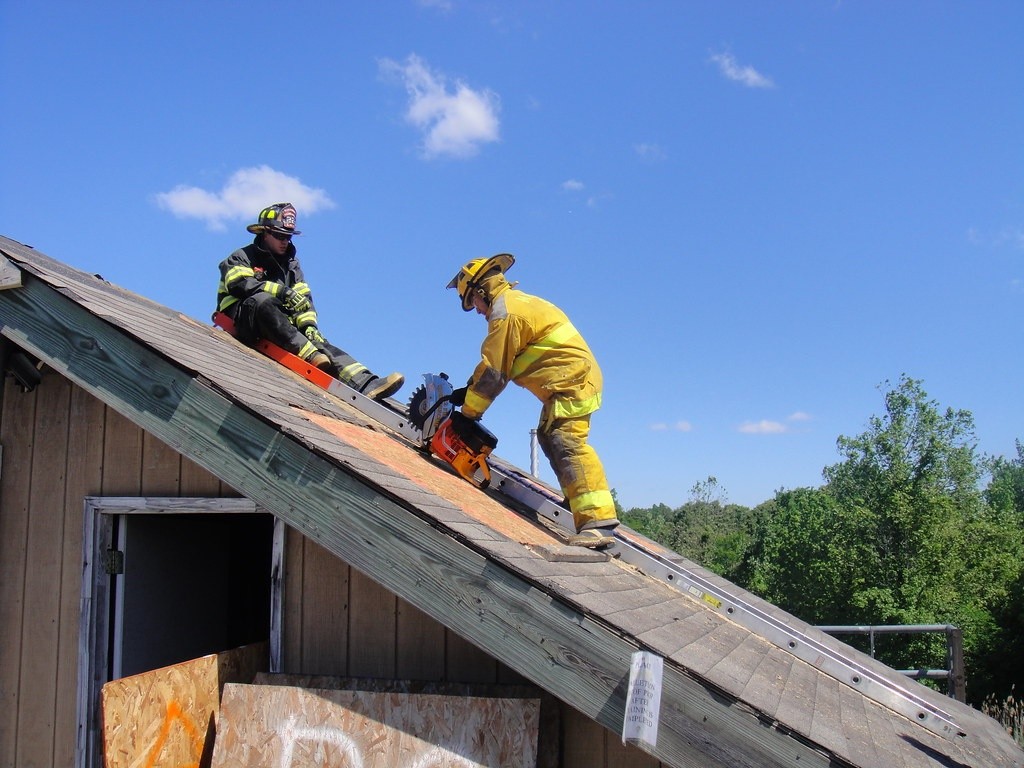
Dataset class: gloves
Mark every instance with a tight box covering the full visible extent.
[281,286,310,313]
[301,324,329,347]
[449,384,469,407]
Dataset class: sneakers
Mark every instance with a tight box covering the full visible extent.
[569,525,615,547]
[360,373,405,401]
[304,349,331,371]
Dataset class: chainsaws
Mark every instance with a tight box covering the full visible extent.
[405,371,498,489]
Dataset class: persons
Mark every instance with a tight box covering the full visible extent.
[216,202,404,400]
[456,253,618,550]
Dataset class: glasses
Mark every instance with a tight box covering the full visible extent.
[266,230,292,242]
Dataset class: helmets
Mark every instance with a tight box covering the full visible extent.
[446,253,515,312]
[246,203,301,235]
